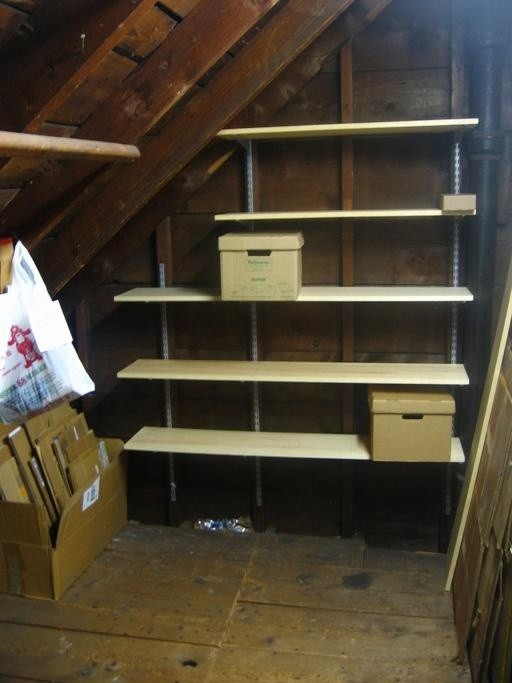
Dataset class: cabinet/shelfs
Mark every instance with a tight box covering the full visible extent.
[114,119,480,465]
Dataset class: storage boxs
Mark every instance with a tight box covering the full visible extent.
[219,231,304,302]
[0,439,128,601]
[368,388,455,463]
